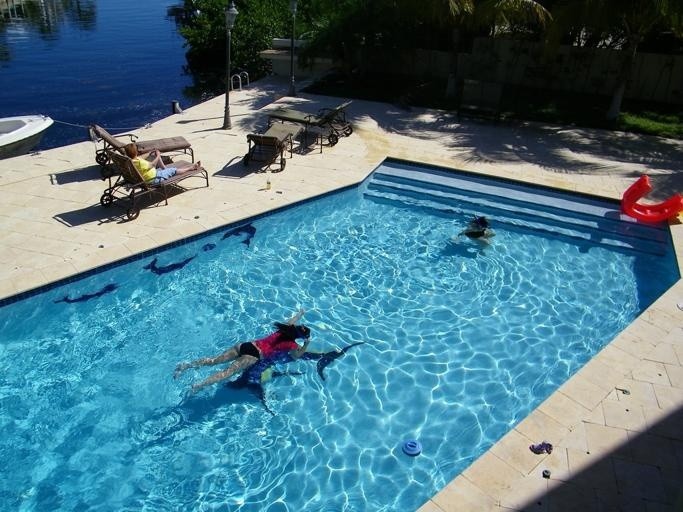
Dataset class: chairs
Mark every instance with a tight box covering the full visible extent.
[245,121,301,170]
[91,124,194,177]
[181,184,189,185]
[265,99,357,145]
[98,151,209,220]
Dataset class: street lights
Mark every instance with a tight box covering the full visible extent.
[288,0,298,98]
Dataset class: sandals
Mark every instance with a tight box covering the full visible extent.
[531,442,552,454]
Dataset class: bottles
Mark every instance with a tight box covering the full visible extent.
[267,177,271,190]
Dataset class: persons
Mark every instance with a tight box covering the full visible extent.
[173,307,311,395]
[458,217,496,245]
[125,144,201,185]
